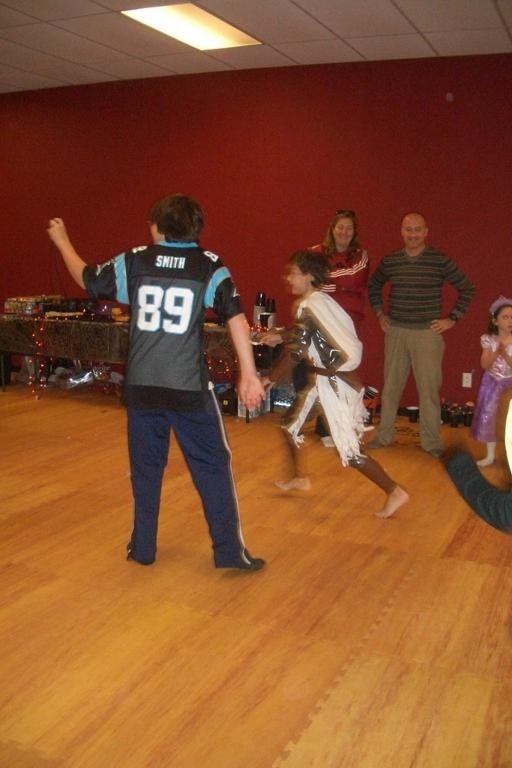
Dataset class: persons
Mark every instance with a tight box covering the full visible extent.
[363,211,476,459]
[308,208,375,450]
[259,248,411,520]
[432,398,511,535]
[43,193,268,573]
[466,294,512,469]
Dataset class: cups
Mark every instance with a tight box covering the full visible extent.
[96,303,111,322]
[406,406,419,423]
[260,313,272,329]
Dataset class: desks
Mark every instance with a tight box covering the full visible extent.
[0,310,288,424]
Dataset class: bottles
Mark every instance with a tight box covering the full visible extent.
[60,297,93,312]
[264,299,276,330]
[38,295,48,317]
[441,402,473,428]
[253,291,266,326]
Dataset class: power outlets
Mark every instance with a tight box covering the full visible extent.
[462,371,474,388]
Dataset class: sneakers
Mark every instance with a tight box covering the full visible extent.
[127,543,157,565]
[214,559,264,570]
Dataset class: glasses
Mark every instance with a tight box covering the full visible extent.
[336,210,354,215]
